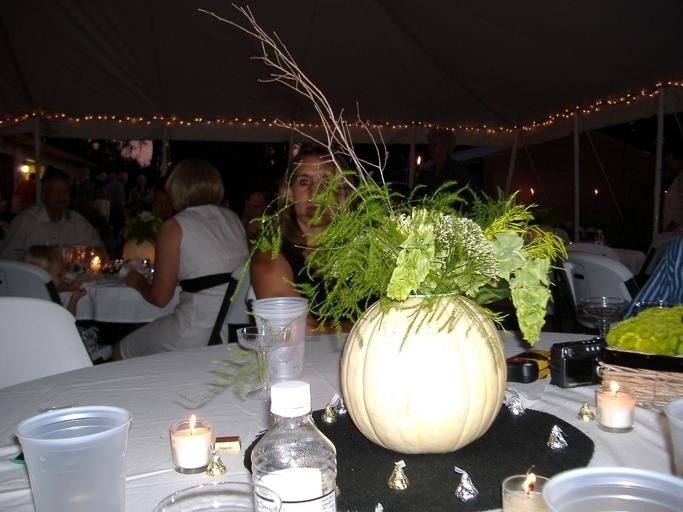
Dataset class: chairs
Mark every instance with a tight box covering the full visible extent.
[0,258,62,309]
[0,293,94,396]
[546,218,676,333]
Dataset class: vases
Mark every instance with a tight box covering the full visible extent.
[341,293,510,455]
[121,237,157,266]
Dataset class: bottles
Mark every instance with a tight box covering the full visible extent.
[250,378,339,512]
[111,256,150,275]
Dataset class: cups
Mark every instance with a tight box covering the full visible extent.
[236,328,294,404]
[250,295,311,380]
[498,471,553,511]
[11,404,133,512]
[664,398,683,483]
[153,480,287,511]
[57,243,105,275]
[542,466,683,511]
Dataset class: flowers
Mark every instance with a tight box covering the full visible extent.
[172,0,571,406]
[120,209,164,247]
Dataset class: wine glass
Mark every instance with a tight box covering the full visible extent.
[578,296,630,342]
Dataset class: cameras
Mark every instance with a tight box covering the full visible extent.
[552,337,602,388]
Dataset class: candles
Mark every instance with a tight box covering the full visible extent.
[499,475,551,512]
